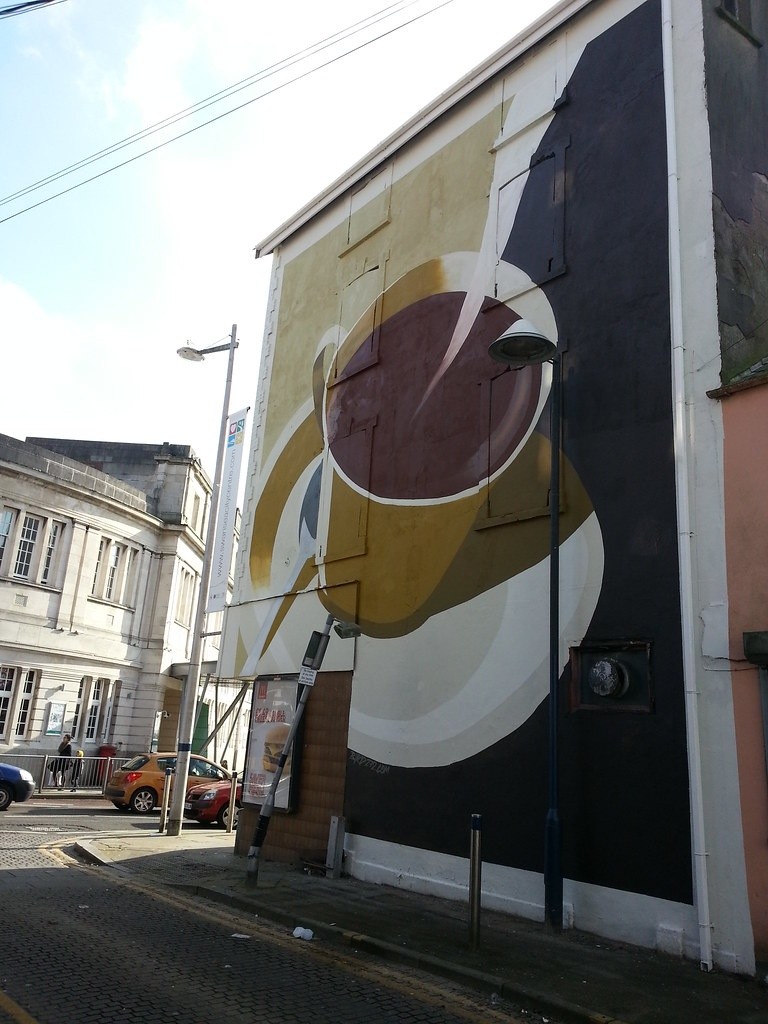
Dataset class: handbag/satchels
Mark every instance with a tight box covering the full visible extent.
[48,771,62,787]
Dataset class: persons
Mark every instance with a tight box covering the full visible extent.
[47,734,72,791]
[70,750,84,792]
[189,760,199,775]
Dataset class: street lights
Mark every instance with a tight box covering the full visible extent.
[245,613,362,887]
[490,316,565,940]
[165,322,240,838]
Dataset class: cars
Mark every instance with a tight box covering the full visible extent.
[105,752,233,815]
[0,763,36,810]
[183,771,244,830]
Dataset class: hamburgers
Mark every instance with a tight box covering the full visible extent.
[263,725,291,775]
